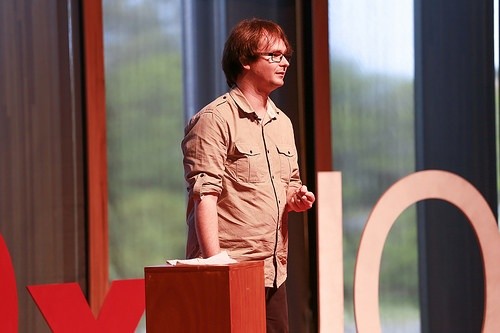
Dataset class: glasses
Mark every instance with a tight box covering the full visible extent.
[255,51,293,62]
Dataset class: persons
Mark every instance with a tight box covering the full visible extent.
[181,16,315,333]
